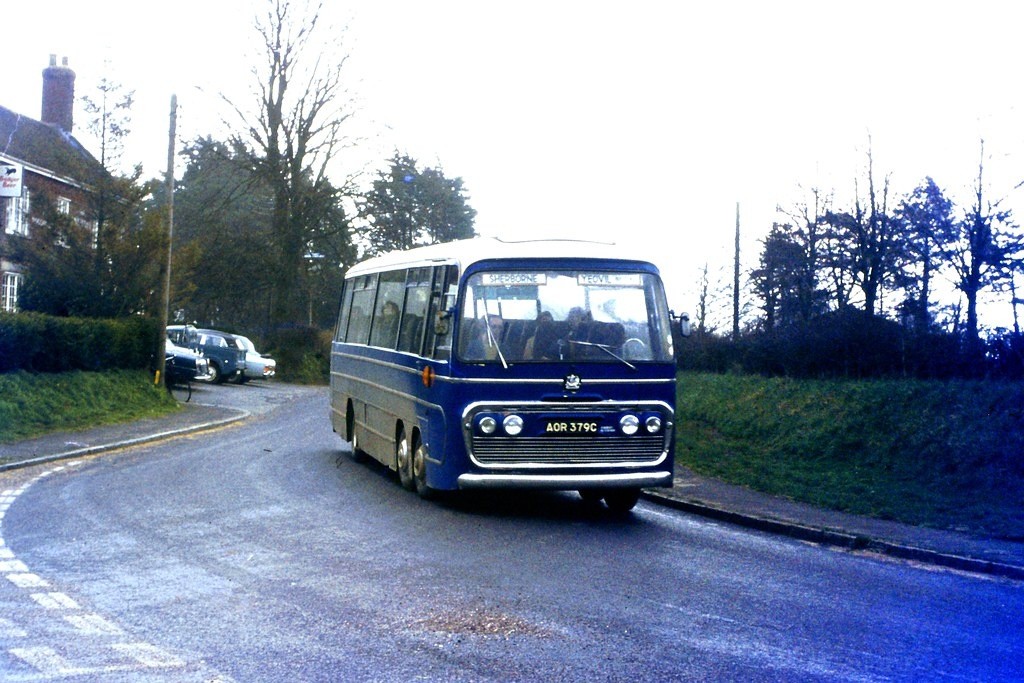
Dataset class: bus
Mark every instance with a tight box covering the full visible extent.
[329,229,692,513]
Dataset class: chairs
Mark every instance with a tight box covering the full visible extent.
[356,311,625,359]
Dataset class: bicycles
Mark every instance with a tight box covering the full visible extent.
[150,353,192,404]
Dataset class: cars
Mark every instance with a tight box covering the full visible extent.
[165,322,278,384]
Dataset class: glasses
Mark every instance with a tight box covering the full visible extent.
[489,323,505,330]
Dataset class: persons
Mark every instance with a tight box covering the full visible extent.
[541,307,594,362]
[464,315,513,361]
[521,310,555,360]
[380,300,400,317]
[347,306,365,320]
